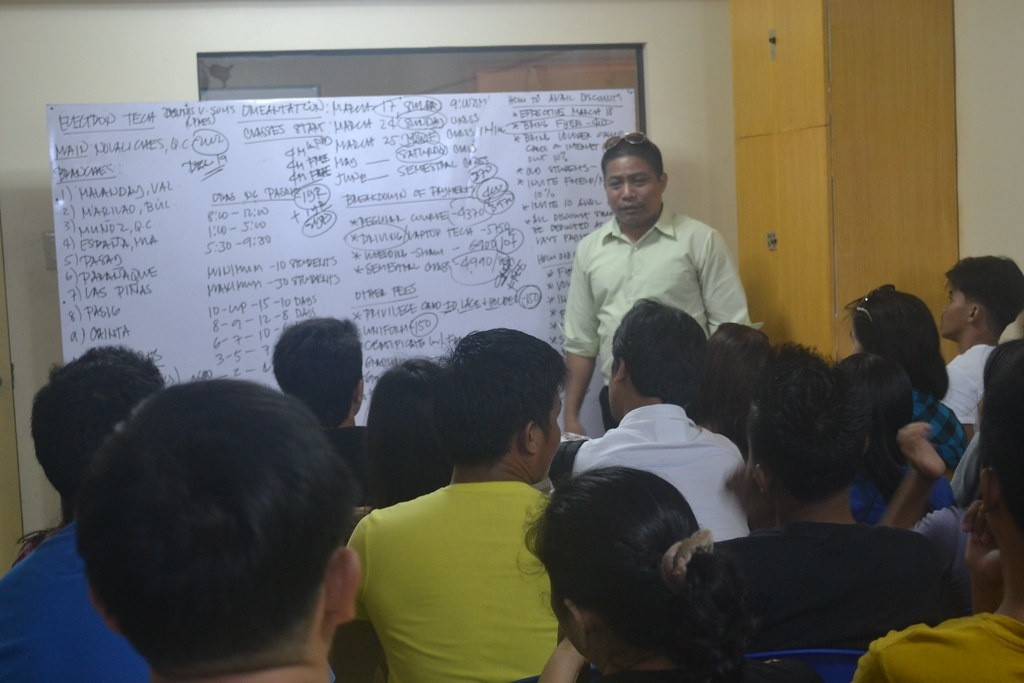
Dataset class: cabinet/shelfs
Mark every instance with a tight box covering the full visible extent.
[730,1,959,369]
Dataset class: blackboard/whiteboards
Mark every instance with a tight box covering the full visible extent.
[44,87,642,436]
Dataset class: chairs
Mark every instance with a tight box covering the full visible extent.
[743,649,867,683]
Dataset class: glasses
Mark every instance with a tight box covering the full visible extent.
[602,131,657,155]
[855,283,897,324]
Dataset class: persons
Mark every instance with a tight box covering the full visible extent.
[348,325,570,683]
[273,317,368,543]
[879,422,968,608]
[837,351,956,525]
[850,403,1024,683]
[564,131,763,440]
[367,359,453,505]
[527,463,819,683]
[687,323,773,463]
[76,378,361,682]
[848,285,967,469]
[0,346,165,683]
[949,339,1024,509]
[572,299,751,542]
[941,256,1024,432]
[713,341,951,683]
